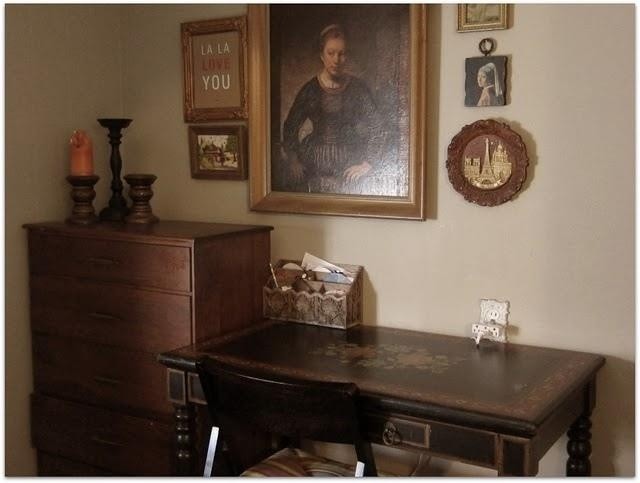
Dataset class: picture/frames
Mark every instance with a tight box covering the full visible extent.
[446,119,529,207]
[189,124,248,180]
[247,3,429,221]
[181,15,249,123]
[457,3,508,33]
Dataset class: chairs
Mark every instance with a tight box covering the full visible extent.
[195,354,431,477]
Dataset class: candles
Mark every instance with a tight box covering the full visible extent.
[69,129,93,177]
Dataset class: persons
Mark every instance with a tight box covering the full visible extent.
[473,64,505,106]
[281,21,387,195]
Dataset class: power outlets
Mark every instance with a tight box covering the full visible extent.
[479,299,509,343]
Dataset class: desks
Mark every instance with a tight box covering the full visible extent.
[157,319,606,477]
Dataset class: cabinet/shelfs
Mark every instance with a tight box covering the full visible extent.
[21,215,274,476]
